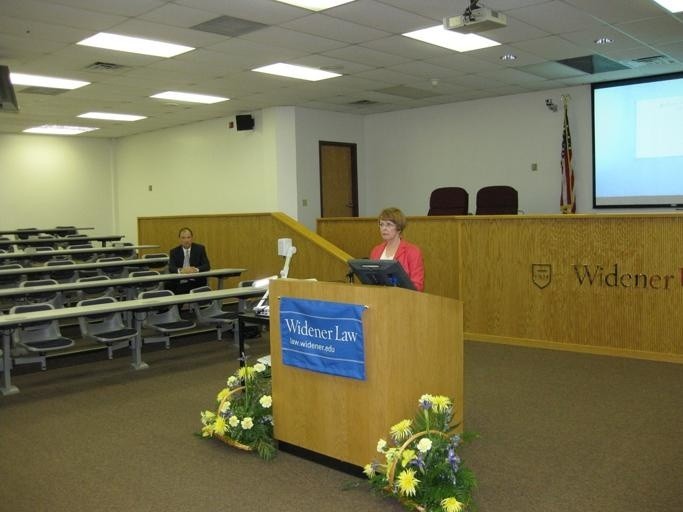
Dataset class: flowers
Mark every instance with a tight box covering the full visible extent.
[192,349,277,460]
[340,393,483,512]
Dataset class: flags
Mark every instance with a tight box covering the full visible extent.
[560,104,576,214]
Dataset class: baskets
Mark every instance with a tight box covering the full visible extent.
[212,384,263,452]
[383,429,469,512]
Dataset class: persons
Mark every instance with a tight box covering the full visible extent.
[369,206,424,292]
[160,226,212,314]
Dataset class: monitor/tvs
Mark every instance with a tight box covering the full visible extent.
[348,260,417,291]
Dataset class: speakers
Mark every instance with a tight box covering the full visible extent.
[236,115,252,130]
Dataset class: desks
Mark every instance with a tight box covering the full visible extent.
[238,312,269,393]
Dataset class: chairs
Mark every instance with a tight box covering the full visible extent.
[475,185,518,214]
[427,187,468,215]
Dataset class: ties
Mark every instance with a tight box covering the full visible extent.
[183,249,190,267]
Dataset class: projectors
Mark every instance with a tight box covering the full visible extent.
[447,7,508,34]
